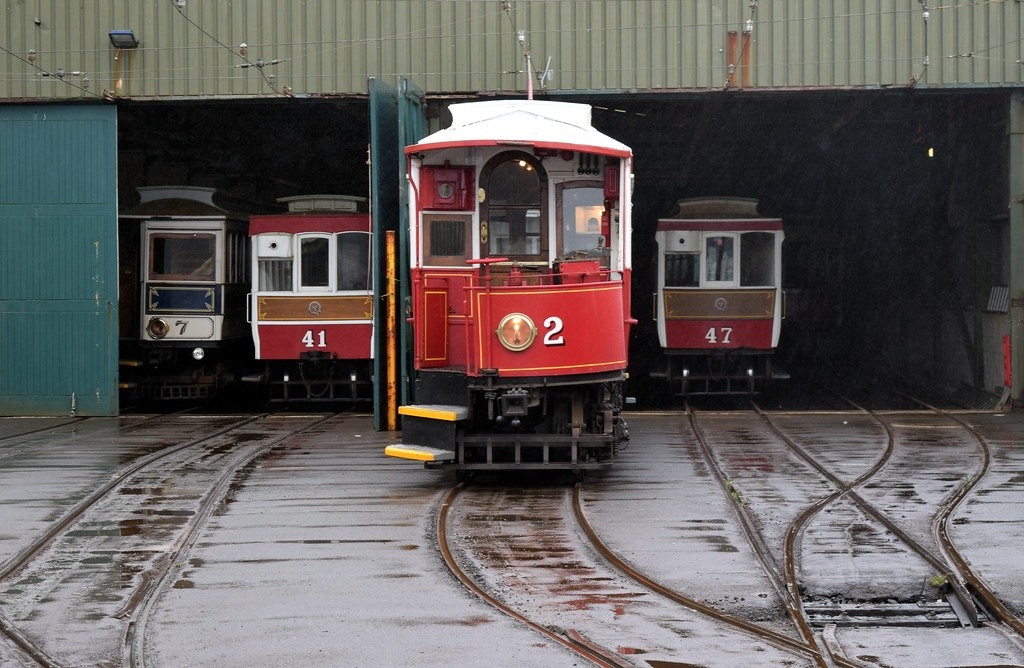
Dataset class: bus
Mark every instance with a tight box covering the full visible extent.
[386,96,637,476]
[116,181,254,413]
[243,191,374,410]
[651,199,791,409]
